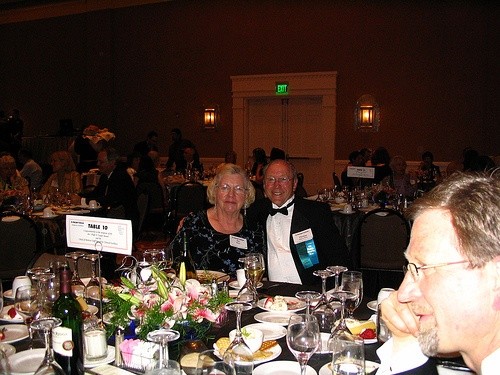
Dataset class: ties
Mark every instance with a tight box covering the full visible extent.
[188,163,190,169]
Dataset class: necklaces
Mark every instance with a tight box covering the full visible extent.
[55,176,66,192]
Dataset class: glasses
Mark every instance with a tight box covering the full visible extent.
[264,176,293,183]
[217,184,248,193]
[403,259,469,281]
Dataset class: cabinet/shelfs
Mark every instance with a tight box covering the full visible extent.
[200,104,219,133]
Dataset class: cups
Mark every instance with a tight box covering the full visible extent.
[230,326,263,354]
[332,334,364,375]
[84,330,106,359]
[143,249,164,261]
[0,181,101,223]
[312,178,423,215]
[236,268,247,286]
[12,276,32,295]
[377,288,396,310]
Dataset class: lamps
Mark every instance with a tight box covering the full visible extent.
[354,95,379,134]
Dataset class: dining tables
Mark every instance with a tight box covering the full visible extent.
[0,280,476,375]
[305,190,413,245]
[82,167,136,196]
[0,196,102,255]
[164,170,255,235]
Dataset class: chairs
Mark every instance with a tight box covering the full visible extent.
[351,208,411,290]
[0,212,39,290]
[175,181,206,228]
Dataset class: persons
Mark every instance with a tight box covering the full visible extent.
[14,148,45,191]
[256,147,286,179]
[341,146,393,189]
[68,124,100,172]
[461,146,500,174]
[248,148,267,176]
[0,154,31,203]
[175,159,340,290]
[165,128,201,172]
[415,151,442,193]
[375,170,500,375]
[125,130,164,207]
[0,109,24,153]
[383,155,414,199]
[55,146,139,220]
[223,151,238,164]
[175,147,204,172]
[37,150,83,198]
[161,163,266,280]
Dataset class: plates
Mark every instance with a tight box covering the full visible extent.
[196,269,228,287]
[242,322,287,340]
[335,319,379,344]
[0,348,47,375]
[83,344,116,368]
[229,280,263,288]
[215,336,282,365]
[257,295,307,312]
[252,360,317,375]
[296,330,341,356]
[5,287,38,299]
[253,311,302,326]
[0,304,41,323]
[367,300,377,312]
[319,358,379,375]
[86,305,98,314]
[0,324,29,344]
[84,365,137,375]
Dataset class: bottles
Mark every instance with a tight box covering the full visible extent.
[171,228,197,282]
[52,265,83,375]
[203,168,217,181]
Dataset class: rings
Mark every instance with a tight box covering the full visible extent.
[395,303,407,313]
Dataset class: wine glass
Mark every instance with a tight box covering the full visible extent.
[197,350,235,375]
[342,270,363,319]
[15,285,40,324]
[84,254,103,298]
[245,253,264,292]
[312,270,334,309]
[295,290,321,351]
[65,250,85,299]
[224,301,254,375]
[36,273,57,308]
[147,329,181,375]
[329,292,356,351]
[326,266,347,314]
[30,318,63,375]
[287,315,319,375]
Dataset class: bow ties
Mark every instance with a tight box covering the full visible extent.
[269,198,295,216]
